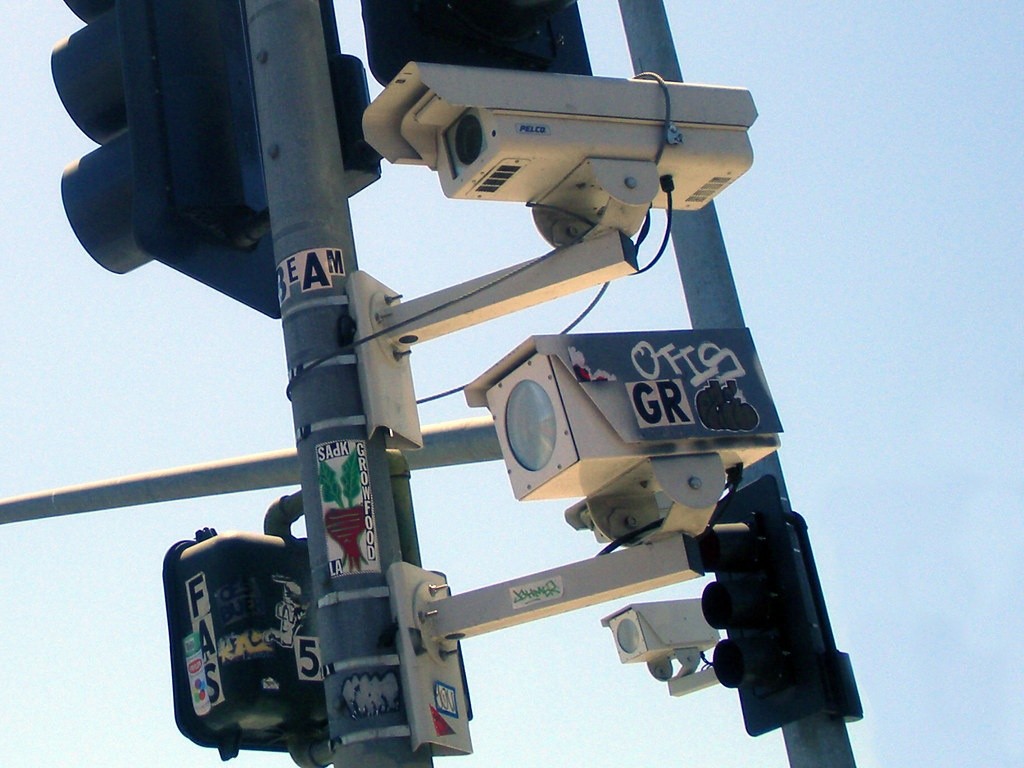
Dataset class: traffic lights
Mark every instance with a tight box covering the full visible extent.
[48,0,280,320]
[364,1,594,90]
[680,474,829,738]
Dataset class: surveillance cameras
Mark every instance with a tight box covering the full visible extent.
[601,598,719,664]
[362,62,758,210]
[462,327,784,501]
